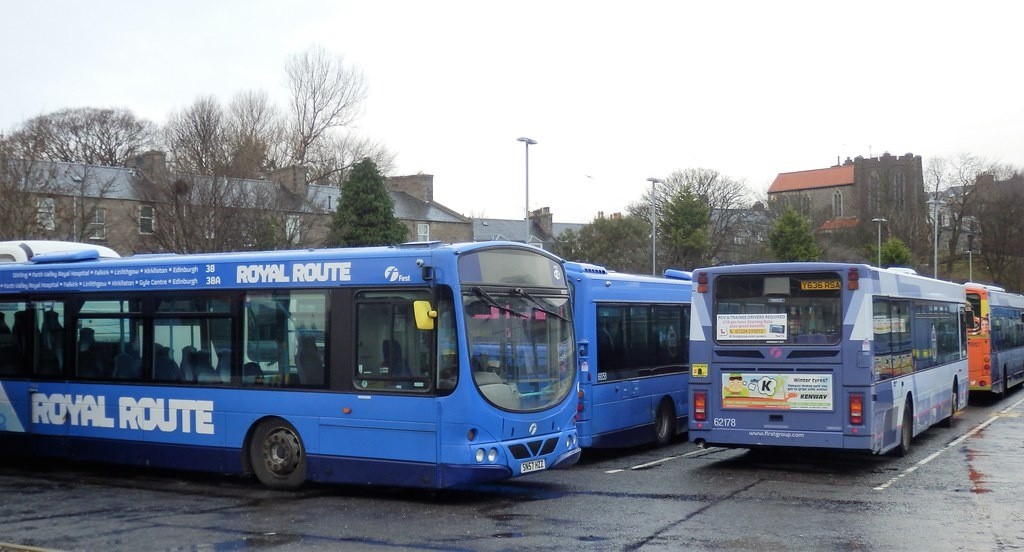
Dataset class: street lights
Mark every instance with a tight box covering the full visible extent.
[924,199,946,279]
[517,136,538,245]
[647,177,663,275]
[966,249,973,282]
[871,218,888,267]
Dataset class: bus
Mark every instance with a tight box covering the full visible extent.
[439,260,691,451]
[1,237,587,495]
[932,280,1024,399]
[0,240,218,376]
[686,260,975,457]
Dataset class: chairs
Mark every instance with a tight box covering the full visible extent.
[787,332,839,344]
[294,335,325,386]
[116,343,234,387]
[381,340,417,391]
